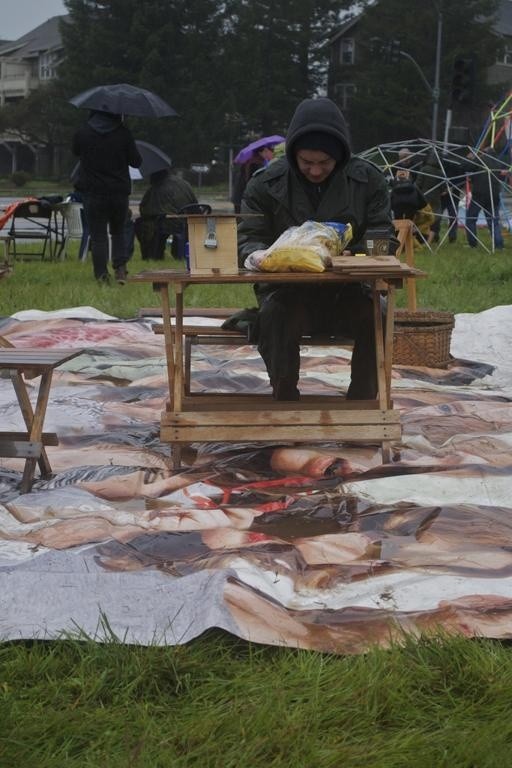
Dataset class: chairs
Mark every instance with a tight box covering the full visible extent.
[36,194,72,260]
[7,201,62,262]
[138,204,212,258]
[55,202,89,262]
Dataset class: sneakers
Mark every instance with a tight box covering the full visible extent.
[115,270,128,285]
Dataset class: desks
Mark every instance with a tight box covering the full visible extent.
[125,257,426,463]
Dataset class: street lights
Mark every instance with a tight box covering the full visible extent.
[364,0,444,141]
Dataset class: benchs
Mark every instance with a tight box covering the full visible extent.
[0,345,85,493]
[140,306,404,445]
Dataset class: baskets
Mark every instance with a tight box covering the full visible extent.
[393,310,456,371]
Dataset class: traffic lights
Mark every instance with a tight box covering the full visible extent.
[210,142,225,167]
[387,37,401,65]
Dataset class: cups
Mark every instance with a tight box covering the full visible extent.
[364,229,394,255]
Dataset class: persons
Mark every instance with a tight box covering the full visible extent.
[139,169,201,260]
[73,110,142,285]
[232,144,268,222]
[389,147,504,250]
[237,98,401,401]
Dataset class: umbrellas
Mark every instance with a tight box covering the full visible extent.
[66,84,180,121]
[134,140,172,176]
[233,135,285,164]
